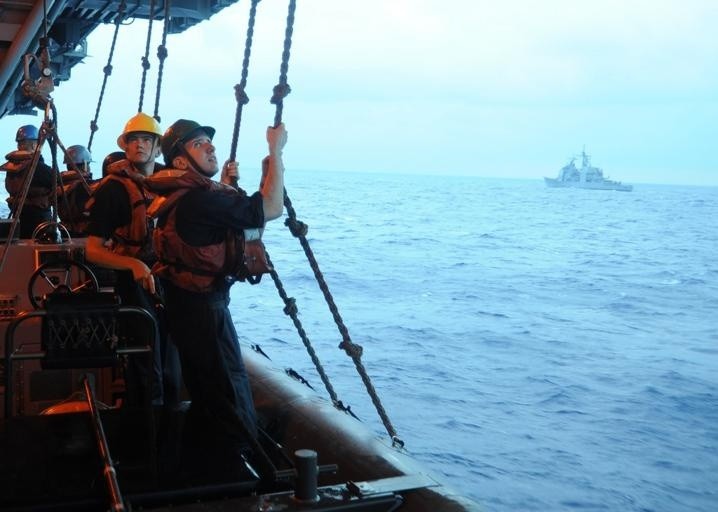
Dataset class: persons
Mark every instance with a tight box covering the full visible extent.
[57,145,98,237]
[85,112,191,420]
[0,125,52,239]
[143,119,288,488]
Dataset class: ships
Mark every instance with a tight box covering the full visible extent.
[542,147,634,193]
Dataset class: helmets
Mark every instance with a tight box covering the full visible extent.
[161,119,216,165]
[63,145,94,164]
[117,112,162,151]
[101,152,127,177]
[15,125,39,142]
